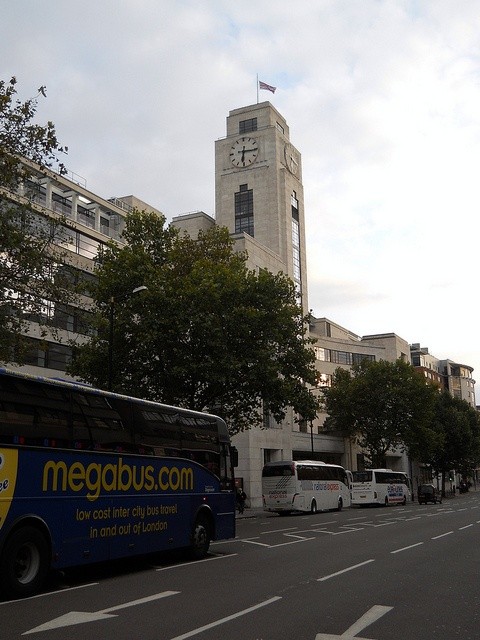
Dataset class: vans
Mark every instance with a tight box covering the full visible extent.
[417,484,444,504]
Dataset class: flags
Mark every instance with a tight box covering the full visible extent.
[260,82,276,94]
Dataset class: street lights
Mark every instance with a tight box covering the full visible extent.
[107,286,148,391]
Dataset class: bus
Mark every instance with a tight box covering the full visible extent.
[352,468,411,507]
[262,460,354,515]
[0,366,238,598]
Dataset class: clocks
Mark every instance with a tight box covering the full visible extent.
[229,136,259,168]
[284,142,299,174]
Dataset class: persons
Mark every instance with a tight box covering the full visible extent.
[236,488,246,514]
